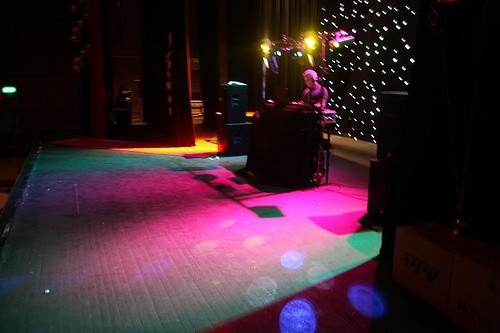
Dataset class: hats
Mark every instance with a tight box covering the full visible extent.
[300,69,319,80]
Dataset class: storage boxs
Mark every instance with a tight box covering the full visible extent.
[443,245,500,333]
[391,217,496,317]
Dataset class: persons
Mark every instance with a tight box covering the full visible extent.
[302,69,328,178]
